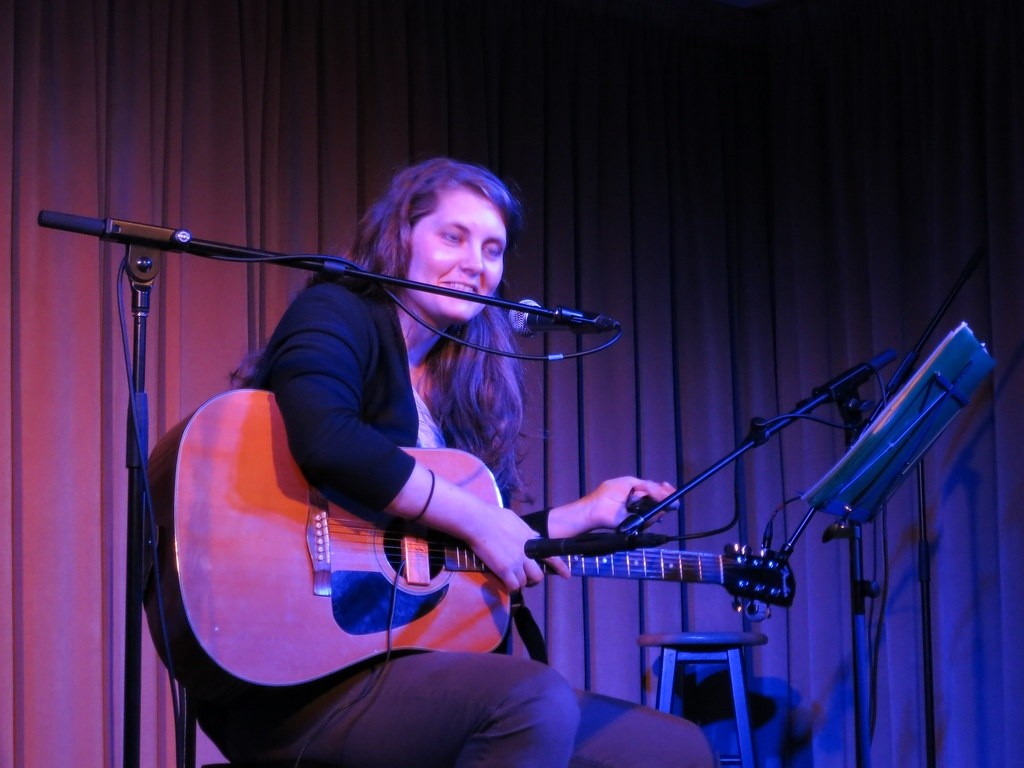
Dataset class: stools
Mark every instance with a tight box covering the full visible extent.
[638,632,769,768]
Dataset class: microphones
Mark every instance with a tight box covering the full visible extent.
[509,300,620,336]
[524,534,668,560]
[745,520,774,621]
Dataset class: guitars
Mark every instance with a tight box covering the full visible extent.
[145,390,794,699]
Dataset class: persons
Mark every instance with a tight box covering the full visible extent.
[200,155,716,768]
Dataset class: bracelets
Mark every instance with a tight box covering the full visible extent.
[412,469,435,523]
